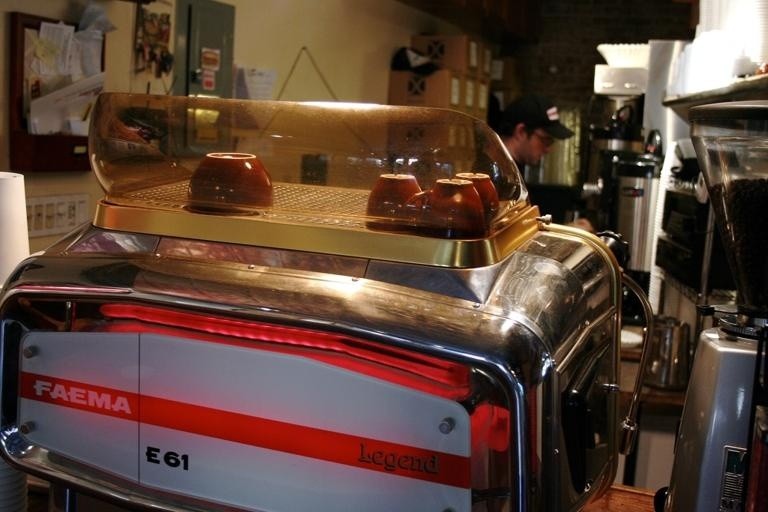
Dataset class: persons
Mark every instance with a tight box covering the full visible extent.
[471,92,598,233]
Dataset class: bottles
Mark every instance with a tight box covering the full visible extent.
[644,317,690,391]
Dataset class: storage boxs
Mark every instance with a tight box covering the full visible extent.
[387,32,494,192]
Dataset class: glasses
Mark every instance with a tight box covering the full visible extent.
[533,130,554,147]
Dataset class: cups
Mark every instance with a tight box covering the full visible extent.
[456,172,501,229]
[188,152,274,214]
[365,172,424,230]
[404,179,486,239]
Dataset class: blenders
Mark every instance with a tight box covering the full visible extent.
[662,99,766,510]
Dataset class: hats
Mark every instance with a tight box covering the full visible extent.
[504,94,574,138]
